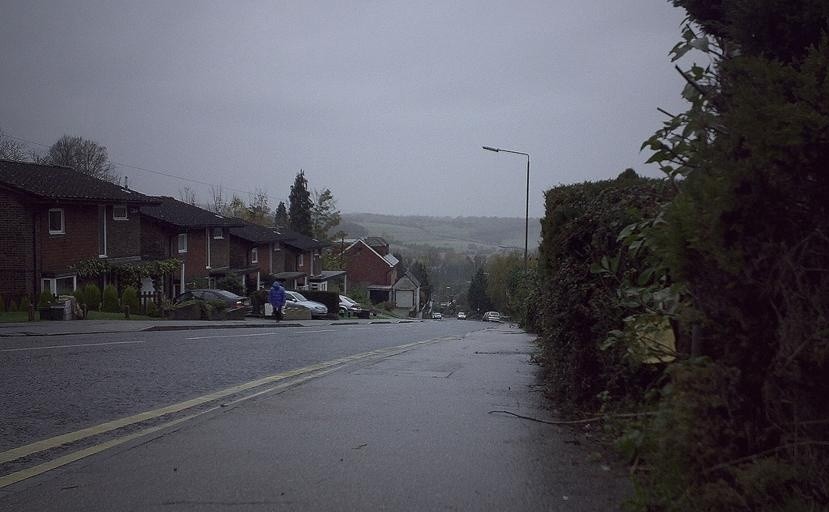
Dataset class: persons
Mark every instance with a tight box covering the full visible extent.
[269,282,286,323]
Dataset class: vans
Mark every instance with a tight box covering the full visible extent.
[482,311,500,322]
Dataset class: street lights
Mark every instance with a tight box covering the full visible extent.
[482,144,530,274]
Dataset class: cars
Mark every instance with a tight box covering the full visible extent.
[432,312,441,319]
[173,288,254,315]
[456,311,466,319]
[339,293,361,317]
[259,290,328,317]
